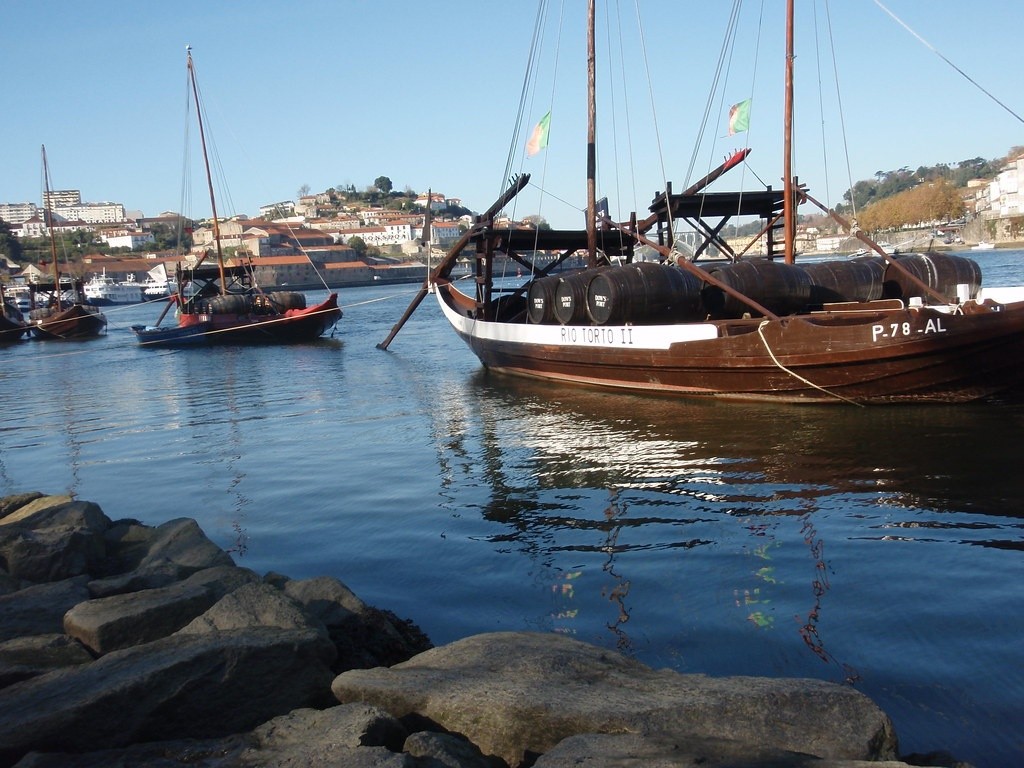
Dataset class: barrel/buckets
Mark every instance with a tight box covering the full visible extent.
[30,308,54,319]
[192,291,307,316]
[525,251,982,323]
[74,305,99,313]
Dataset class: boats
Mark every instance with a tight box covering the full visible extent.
[83,267,144,306]
[847,248,870,261]
[5,278,83,312]
[871,241,899,256]
[970,242,995,251]
[143,282,179,303]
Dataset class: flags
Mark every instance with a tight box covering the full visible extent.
[729,97,752,136]
[148,264,167,284]
[527,112,551,157]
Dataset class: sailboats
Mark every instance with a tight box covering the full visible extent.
[425,0,1024,405]
[0,283,27,341]
[24,144,108,339]
[130,45,343,346]
